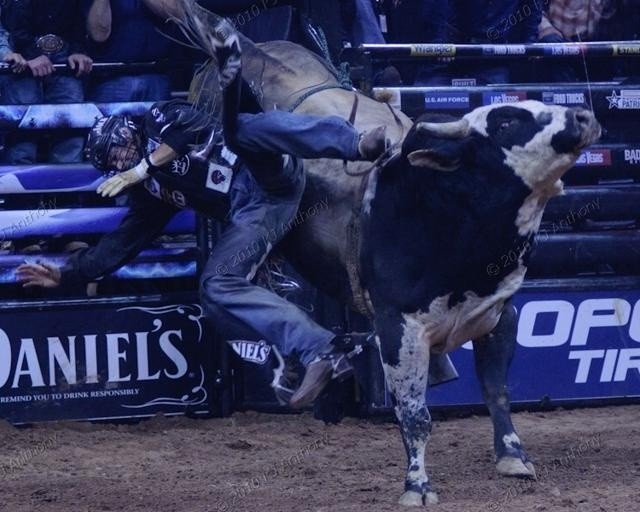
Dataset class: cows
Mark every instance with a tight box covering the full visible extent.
[148,0,603,508]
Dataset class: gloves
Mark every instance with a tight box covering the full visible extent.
[96,158,150,198]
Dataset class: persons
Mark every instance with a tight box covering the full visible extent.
[0,1,639,300]
[17,99,392,409]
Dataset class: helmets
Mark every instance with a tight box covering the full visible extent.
[85,115,144,172]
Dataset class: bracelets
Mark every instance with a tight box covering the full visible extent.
[139,154,159,177]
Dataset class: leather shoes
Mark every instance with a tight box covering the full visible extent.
[289,344,352,410]
[357,125,392,161]
